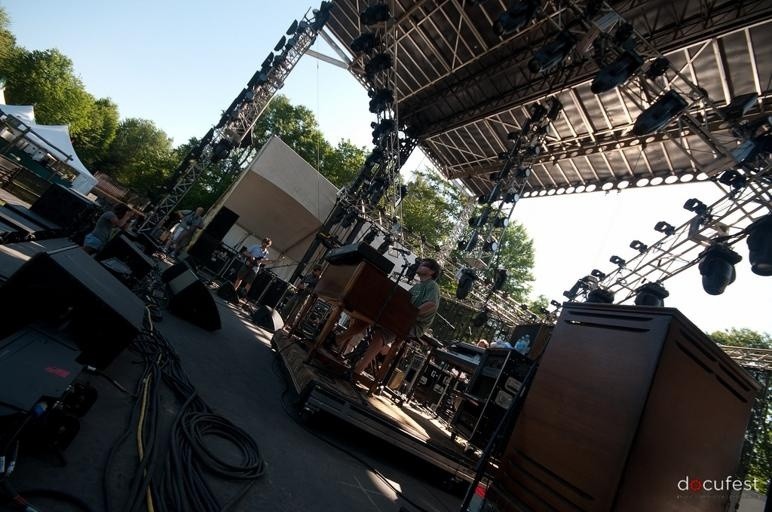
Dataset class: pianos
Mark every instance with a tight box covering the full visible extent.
[324,242,395,276]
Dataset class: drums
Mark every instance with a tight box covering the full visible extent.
[172,223,187,244]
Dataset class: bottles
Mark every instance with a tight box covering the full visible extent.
[515,334,530,355]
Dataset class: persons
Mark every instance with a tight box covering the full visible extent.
[280,263,322,320]
[232,236,272,303]
[162,205,204,259]
[477,338,490,349]
[326,257,443,357]
[82,202,136,256]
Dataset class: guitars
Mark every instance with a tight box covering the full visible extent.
[245,254,285,268]
